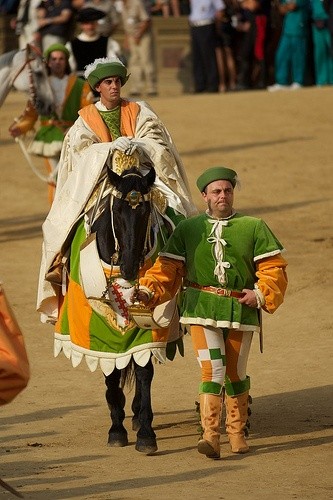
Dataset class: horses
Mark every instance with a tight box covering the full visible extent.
[67,149,178,455]
[0,44,56,117]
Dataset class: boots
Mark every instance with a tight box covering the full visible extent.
[194,382,223,459]
[225,376,252,453]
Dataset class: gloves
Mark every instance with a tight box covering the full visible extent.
[109,136,138,155]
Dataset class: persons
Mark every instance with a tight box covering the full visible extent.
[129,167,287,459]
[9,0,180,184]
[188,0,333,95]
[45,67,189,284]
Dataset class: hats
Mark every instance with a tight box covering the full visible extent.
[74,7,107,22]
[84,56,127,91]
[196,167,237,193]
[44,43,70,59]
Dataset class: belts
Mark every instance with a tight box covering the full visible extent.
[39,120,75,127]
[186,281,246,299]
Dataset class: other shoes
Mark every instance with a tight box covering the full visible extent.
[266,82,301,91]
[146,92,156,97]
[128,92,141,97]
[46,255,62,284]
